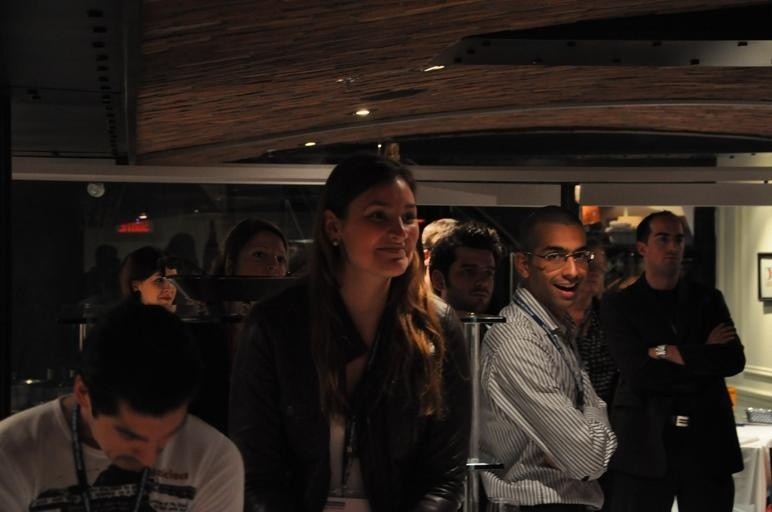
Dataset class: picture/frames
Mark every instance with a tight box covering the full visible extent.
[757,250,772,303]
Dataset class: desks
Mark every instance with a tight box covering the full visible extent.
[671,425,772,512]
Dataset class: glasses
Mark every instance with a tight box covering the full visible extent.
[523,252,595,266]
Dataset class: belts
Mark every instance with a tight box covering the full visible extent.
[672,415,690,427]
